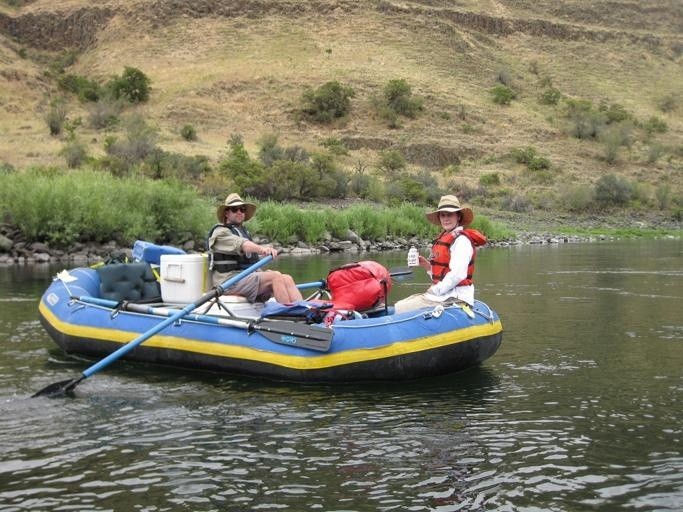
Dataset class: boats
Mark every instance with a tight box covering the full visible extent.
[38,256,503,384]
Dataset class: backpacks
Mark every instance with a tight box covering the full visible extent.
[327,261,392,311]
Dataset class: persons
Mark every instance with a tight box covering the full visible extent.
[208,193,303,307]
[394,195,487,314]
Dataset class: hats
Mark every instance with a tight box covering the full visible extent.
[217,193,257,223]
[425,195,474,226]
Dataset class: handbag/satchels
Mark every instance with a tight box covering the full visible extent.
[261,301,333,317]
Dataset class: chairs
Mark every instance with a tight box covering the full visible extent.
[160,253,252,304]
[98,262,161,304]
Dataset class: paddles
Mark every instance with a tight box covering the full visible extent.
[69,293,334,355]
[30,248,285,400]
[295,266,415,290]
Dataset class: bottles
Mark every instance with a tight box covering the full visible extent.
[348,307,354,320]
[407,244,420,268]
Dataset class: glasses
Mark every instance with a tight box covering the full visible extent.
[228,207,247,213]
